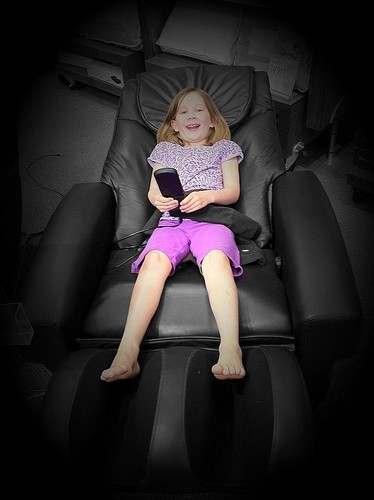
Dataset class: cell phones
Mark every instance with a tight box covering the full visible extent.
[153,168,185,216]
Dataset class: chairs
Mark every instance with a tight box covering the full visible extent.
[19,67,364,499]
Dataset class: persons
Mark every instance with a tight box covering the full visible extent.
[100,88,246,381]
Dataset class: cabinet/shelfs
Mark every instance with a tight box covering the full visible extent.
[53,0,356,131]
